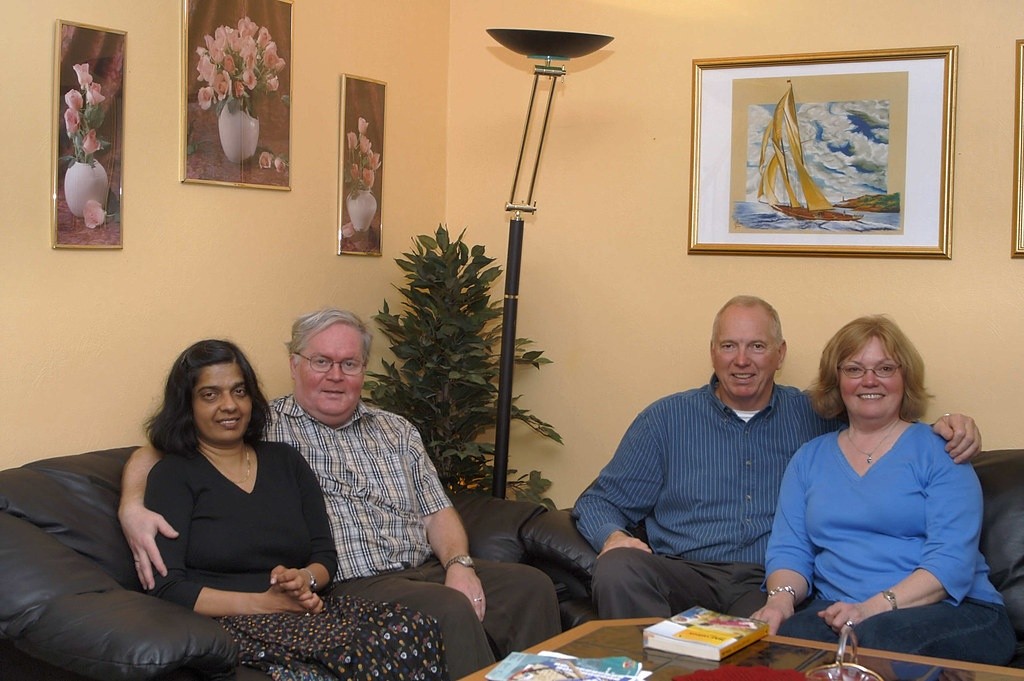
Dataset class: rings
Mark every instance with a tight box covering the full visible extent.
[844,621,855,628]
[472,598,482,602]
[134,559,139,562]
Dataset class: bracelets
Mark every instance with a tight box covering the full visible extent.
[944,413,950,417]
[767,586,796,602]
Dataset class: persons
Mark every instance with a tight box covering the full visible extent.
[571,294,983,619]
[749,316,1017,669]
[118,306,563,681]
[136,340,452,681]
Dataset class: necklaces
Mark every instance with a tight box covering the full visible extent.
[226,443,250,483]
[847,419,900,463]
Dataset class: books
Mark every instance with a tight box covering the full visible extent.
[485,652,653,681]
[643,606,769,661]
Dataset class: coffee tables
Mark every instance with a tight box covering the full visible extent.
[457,618,1024,681]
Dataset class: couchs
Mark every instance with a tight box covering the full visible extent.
[520,449,1024,669]
[1,447,549,681]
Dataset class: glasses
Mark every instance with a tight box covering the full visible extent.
[837,364,902,379]
[293,351,366,376]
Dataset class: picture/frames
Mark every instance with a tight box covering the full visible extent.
[50,20,128,250]
[181,0,295,190]
[337,72,387,256]
[688,45,959,261]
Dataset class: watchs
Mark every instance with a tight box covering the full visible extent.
[445,554,475,572]
[301,568,316,591]
[880,591,898,611]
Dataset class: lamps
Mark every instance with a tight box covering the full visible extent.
[483,27,615,500]
